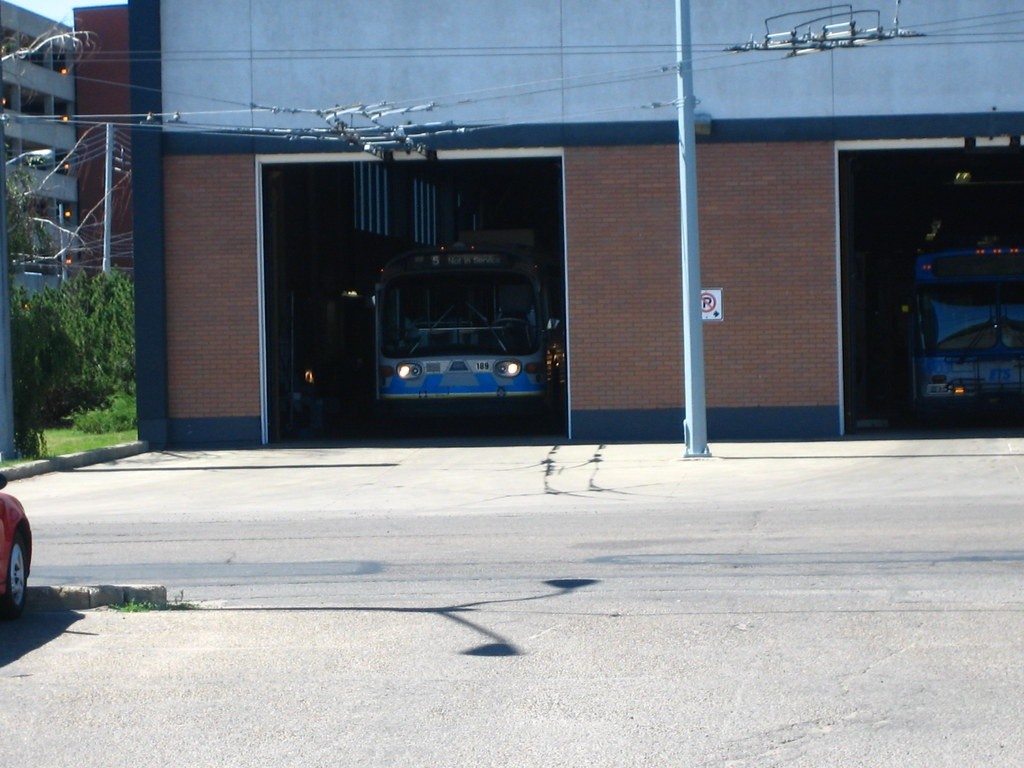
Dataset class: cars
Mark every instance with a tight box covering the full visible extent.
[0,472,33,619]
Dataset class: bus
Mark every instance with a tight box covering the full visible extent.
[368,245,555,424]
[895,242,1024,429]
